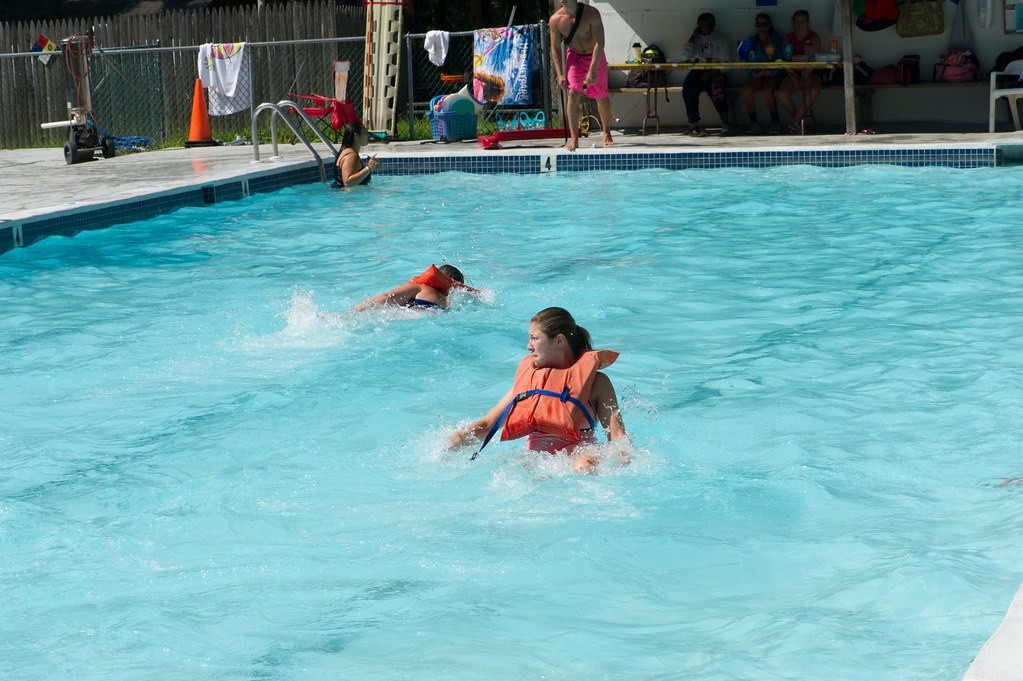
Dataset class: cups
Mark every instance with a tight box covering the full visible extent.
[631,43,641,62]
[805,41,812,57]
[783,42,794,61]
[828,40,838,55]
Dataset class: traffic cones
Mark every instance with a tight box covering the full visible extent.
[184,79,220,148]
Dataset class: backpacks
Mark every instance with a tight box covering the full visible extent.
[626,44,668,88]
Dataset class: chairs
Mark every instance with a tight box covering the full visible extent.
[286,92,361,145]
[988,59,1023,134]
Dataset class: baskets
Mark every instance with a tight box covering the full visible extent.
[429,111,478,142]
[495,111,522,131]
[518,111,546,130]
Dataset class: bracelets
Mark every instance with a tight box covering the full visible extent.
[368,165,372,172]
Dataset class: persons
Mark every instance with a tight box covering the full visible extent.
[331,122,379,188]
[334,265,464,322]
[738,13,784,135]
[440,307,637,475]
[680,13,737,135]
[774,9,822,134]
[548,0,615,151]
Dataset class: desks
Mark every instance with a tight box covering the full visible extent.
[606,61,841,137]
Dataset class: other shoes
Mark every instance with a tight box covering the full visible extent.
[742,123,759,131]
[686,122,706,136]
[788,121,807,135]
[767,123,779,134]
[721,123,738,137]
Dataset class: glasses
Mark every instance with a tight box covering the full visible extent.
[698,20,712,25]
[754,22,770,27]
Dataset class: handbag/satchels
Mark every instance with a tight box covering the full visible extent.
[828,60,872,85]
[897,53,922,87]
[852,0,899,32]
[871,64,898,84]
[933,48,985,84]
[897,0,945,38]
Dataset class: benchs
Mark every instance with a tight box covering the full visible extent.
[606,81,1010,128]
